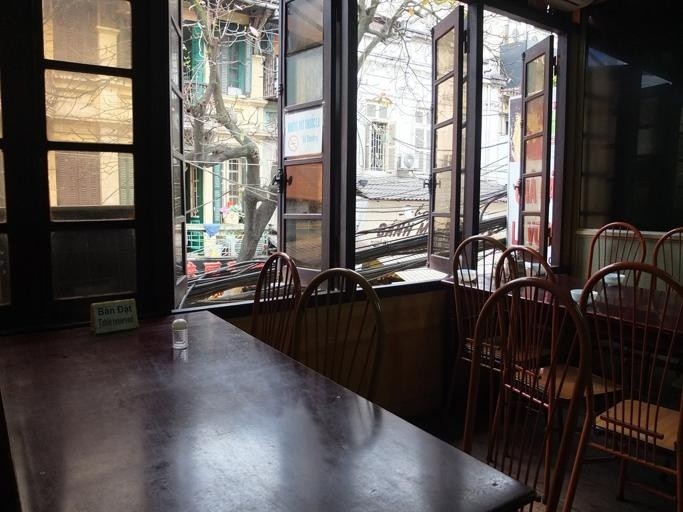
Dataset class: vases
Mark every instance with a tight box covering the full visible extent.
[224,214,239,224]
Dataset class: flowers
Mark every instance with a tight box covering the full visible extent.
[219,200,241,218]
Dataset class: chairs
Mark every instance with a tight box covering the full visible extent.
[295,265,387,403]
[587,222,649,315]
[647,225,682,313]
[251,250,302,361]
[559,258,681,511]
[450,233,552,469]
[458,275,595,512]
[493,242,630,503]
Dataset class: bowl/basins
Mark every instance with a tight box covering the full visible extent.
[603,274,626,287]
[571,288,598,306]
[529,262,551,275]
[457,269,477,282]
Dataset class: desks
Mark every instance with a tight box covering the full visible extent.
[441,271,683,378]
[0,311,538,512]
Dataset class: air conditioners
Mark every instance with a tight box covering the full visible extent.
[397,151,419,170]
[230,238,264,257]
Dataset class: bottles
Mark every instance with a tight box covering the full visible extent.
[172,319,189,350]
